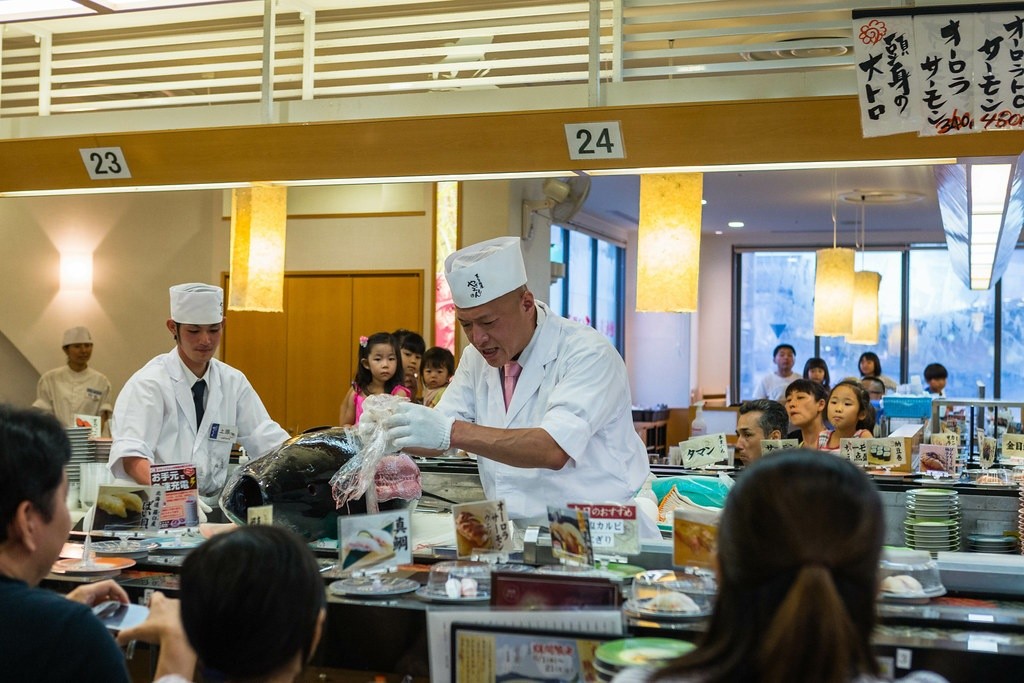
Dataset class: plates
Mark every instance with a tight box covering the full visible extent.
[962,469,1005,480]
[593,562,647,580]
[913,479,961,488]
[624,595,715,619]
[1017,478,1024,556]
[589,636,700,683]
[682,470,719,476]
[415,582,491,602]
[964,534,1019,554]
[328,576,421,596]
[915,472,960,479]
[865,470,912,481]
[661,572,724,596]
[52,557,136,575]
[66,427,112,482]
[968,481,1017,489]
[876,589,947,604]
[82,541,161,560]
[142,536,208,555]
[697,464,735,471]
[903,489,962,555]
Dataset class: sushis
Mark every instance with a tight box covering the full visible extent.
[346,526,397,555]
[445,576,479,600]
[976,476,1003,483]
[675,522,717,555]
[456,511,489,548]
[879,575,924,594]
[643,592,700,613]
[920,452,948,472]
[98,492,144,519]
[870,444,892,461]
[550,522,588,554]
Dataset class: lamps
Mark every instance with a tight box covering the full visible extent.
[228,186,288,315]
[634,41,703,313]
[840,194,881,344]
[813,170,855,338]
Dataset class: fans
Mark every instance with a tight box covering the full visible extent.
[523,167,592,240]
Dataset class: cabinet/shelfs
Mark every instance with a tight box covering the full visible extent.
[633,418,668,460]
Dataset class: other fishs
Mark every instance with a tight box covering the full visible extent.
[219,426,422,545]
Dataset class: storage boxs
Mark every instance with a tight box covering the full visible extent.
[630,407,670,422]
[882,393,939,419]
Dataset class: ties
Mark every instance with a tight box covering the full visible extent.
[502,360,521,412]
[190,379,206,432]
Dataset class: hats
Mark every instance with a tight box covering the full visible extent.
[442,235,527,308]
[168,282,223,323]
[61,326,93,345]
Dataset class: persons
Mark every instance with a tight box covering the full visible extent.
[734,400,789,468]
[179,526,328,683]
[341,329,426,425]
[35,326,116,441]
[343,332,412,428]
[811,380,873,451]
[404,375,425,406]
[359,238,666,544]
[752,344,950,409]
[107,282,294,526]
[419,347,456,410]
[785,380,834,449]
[0,407,203,683]
[610,448,954,683]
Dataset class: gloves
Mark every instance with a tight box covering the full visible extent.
[197,497,212,522]
[383,401,455,450]
[356,408,376,444]
